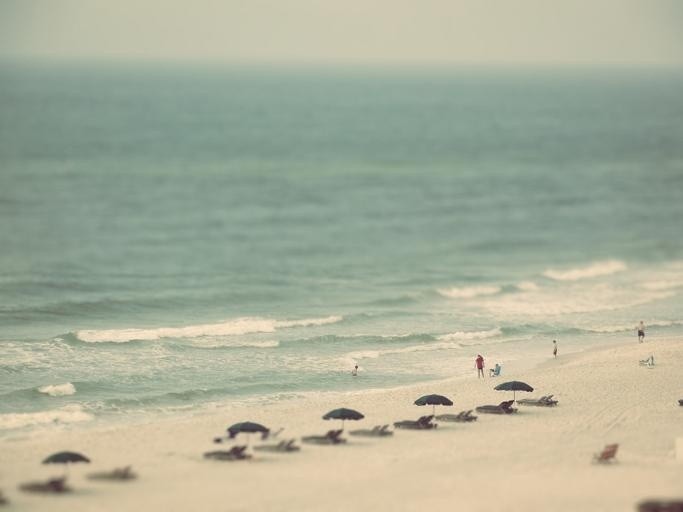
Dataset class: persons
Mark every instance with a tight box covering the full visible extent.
[352,365,359,376]
[638,320,645,343]
[474,354,485,378]
[492,363,501,376]
[552,340,558,357]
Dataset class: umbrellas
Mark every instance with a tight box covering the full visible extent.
[413,393,453,429]
[323,407,365,432]
[494,381,534,406]
[42,451,91,491]
[228,420,270,453]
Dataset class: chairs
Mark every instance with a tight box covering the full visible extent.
[435,409,477,423]
[349,424,393,437]
[475,400,514,414]
[301,429,345,445]
[203,438,300,462]
[393,414,435,429]
[515,394,558,407]
[0,464,135,505]
